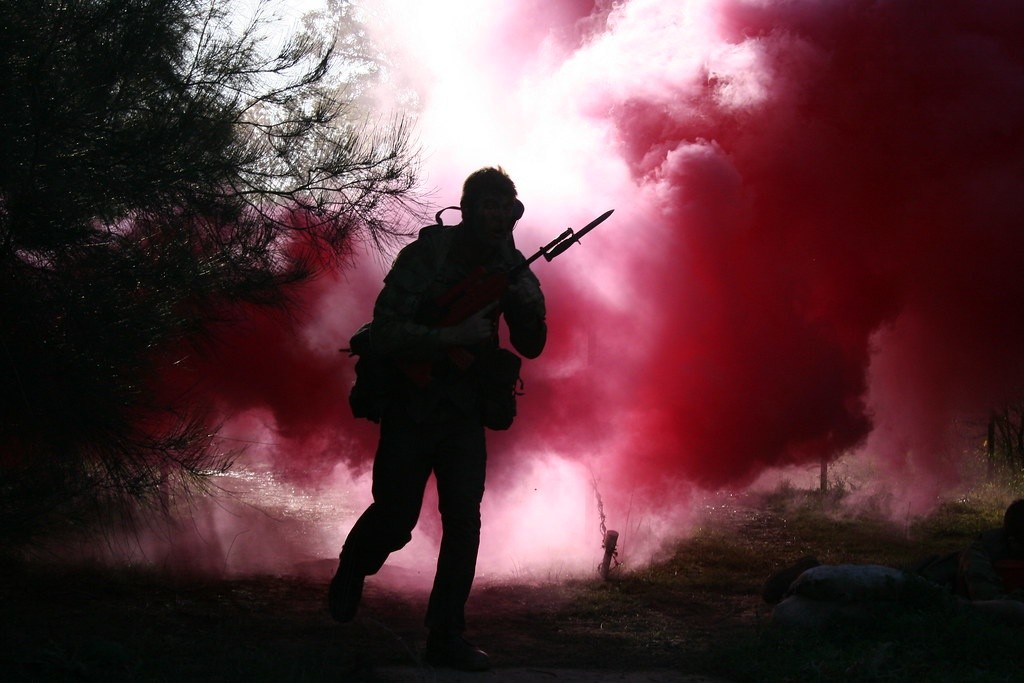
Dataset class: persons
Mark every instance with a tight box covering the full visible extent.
[328,169,547,672]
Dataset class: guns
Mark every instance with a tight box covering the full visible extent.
[396,207,615,386]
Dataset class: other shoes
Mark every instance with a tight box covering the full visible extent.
[328,562,365,623]
[425,632,491,672]
